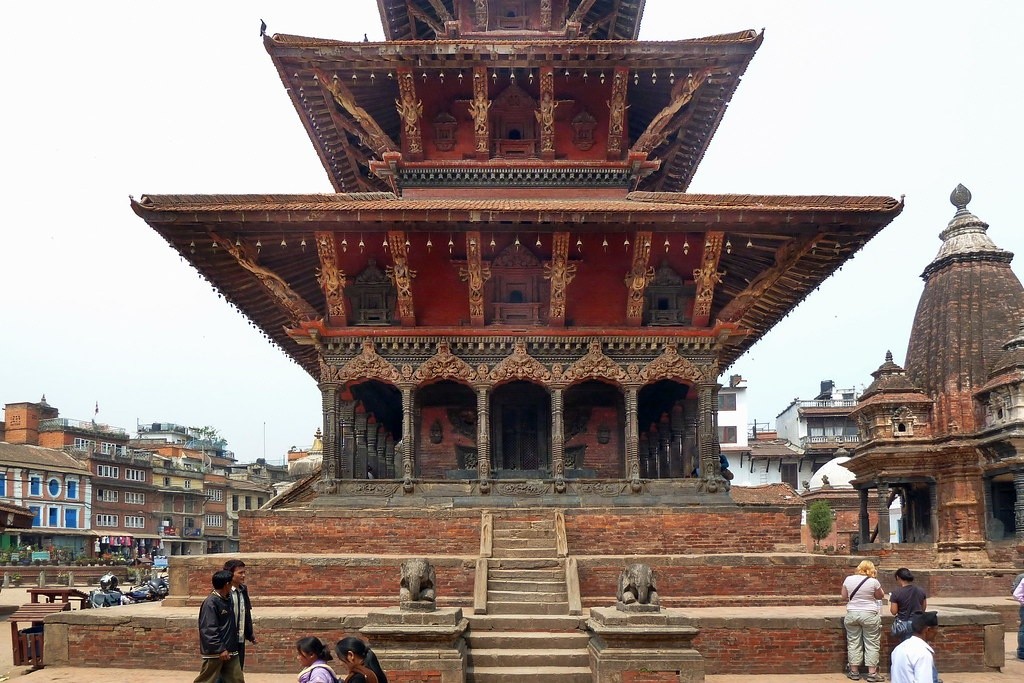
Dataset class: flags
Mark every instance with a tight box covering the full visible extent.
[95,401,98,415]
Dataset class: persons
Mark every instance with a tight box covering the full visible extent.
[892,610,940,683]
[842,560,885,682]
[296,636,337,683]
[193,559,259,683]
[889,568,943,683]
[16,540,31,551]
[334,636,388,683]
[1011,572,1024,660]
[718,444,729,471]
[80,547,85,555]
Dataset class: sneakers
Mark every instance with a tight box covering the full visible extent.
[848,671,859,679]
[867,672,884,682]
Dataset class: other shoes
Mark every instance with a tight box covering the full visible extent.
[1017,657,1024,661]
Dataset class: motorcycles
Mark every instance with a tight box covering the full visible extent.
[124,578,169,602]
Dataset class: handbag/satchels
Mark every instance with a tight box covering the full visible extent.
[721,469,733,480]
[892,620,907,634]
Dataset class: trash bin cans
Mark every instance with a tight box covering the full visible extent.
[19,626,44,658]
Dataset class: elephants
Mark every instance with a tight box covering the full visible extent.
[399,559,436,602]
[616,563,660,605]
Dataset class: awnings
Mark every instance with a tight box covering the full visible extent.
[5,528,239,543]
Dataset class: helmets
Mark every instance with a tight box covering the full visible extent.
[101,574,118,592]
